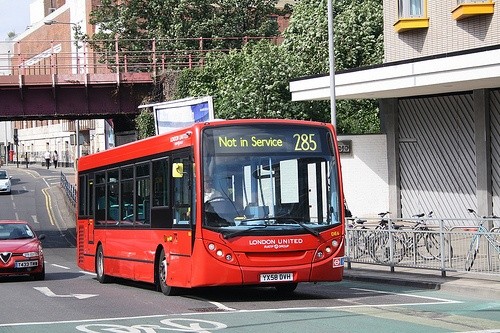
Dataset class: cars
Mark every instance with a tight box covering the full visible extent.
[0,219,46,279]
[0,169,12,194]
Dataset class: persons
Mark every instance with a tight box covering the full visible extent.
[44,150,51,169]
[204,174,222,203]
[53,150,58,168]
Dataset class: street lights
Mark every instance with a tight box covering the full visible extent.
[43,19,79,74]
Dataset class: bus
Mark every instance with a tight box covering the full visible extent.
[73,118,347,296]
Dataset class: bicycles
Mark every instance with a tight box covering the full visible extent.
[344,210,453,266]
[463,208,500,271]
